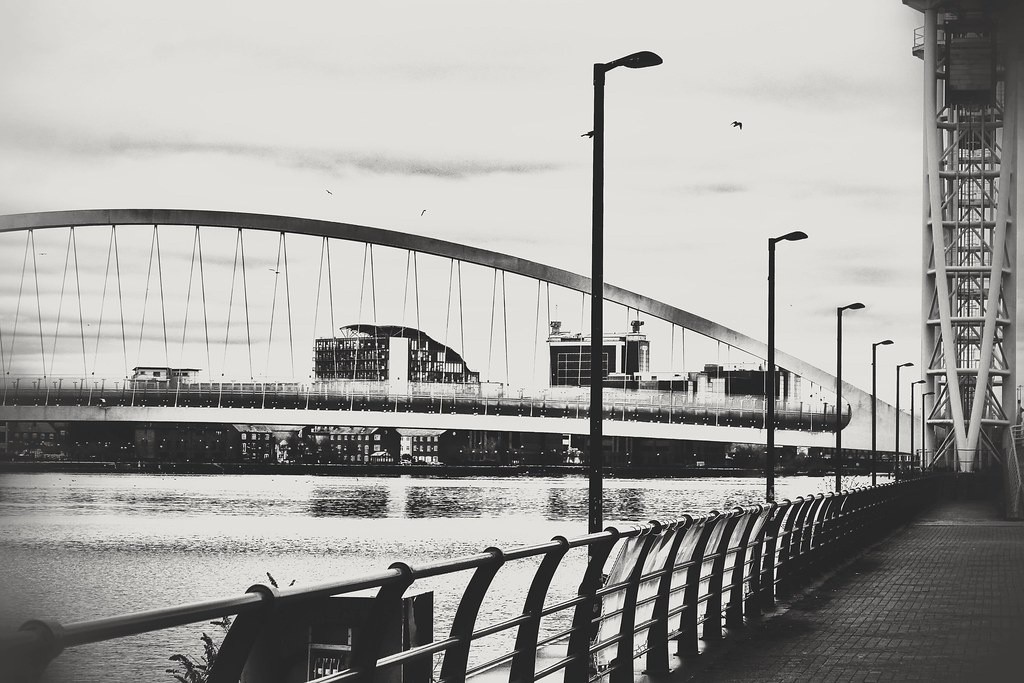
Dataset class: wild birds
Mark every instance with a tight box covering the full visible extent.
[268,268,281,275]
[580,131,594,139]
[325,188,333,196]
[420,210,427,217]
[729,121,743,130]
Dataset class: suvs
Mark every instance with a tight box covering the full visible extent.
[399,460,412,465]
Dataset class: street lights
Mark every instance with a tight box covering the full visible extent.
[766,231,808,501]
[835,303,866,491]
[911,380,926,470]
[588,51,663,561]
[896,362,914,478]
[922,392,935,467]
[872,340,894,485]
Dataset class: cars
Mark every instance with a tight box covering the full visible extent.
[429,461,443,465]
